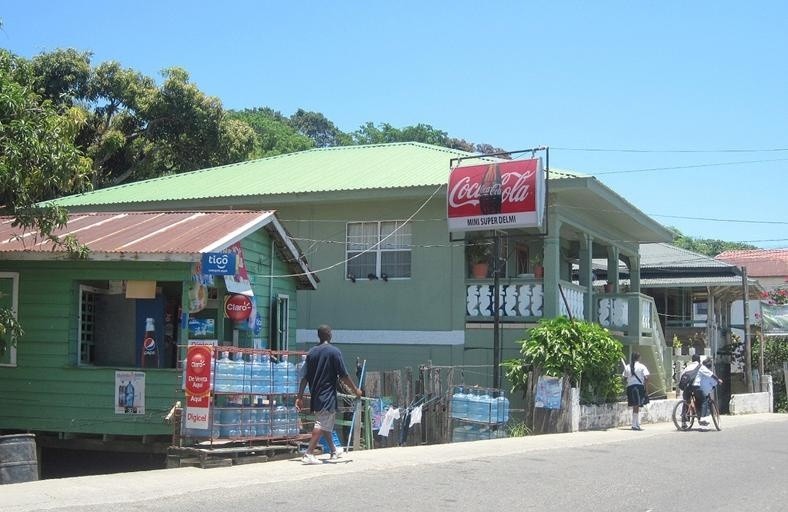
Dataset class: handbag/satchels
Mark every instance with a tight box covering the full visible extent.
[679,363,702,391]
[643,389,650,405]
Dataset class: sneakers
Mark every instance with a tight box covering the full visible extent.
[682,421,688,428]
[329,447,345,459]
[632,426,645,430]
[300,452,323,464]
[698,417,711,424]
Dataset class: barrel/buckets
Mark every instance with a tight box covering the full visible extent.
[182,347,307,438]
[451,387,509,442]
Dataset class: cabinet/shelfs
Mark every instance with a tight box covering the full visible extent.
[172,345,311,454]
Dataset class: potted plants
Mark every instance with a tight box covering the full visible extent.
[530,255,545,279]
[673,334,696,355]
[466,240,494,278]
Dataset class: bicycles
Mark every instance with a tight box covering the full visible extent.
[672,390,721,431]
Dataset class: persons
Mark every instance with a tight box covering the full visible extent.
[679,354,723,428]
[623,351,650,430]
[295,325,363,464]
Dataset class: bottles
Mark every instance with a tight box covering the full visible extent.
[481,164,503,214]
[141,319,160,372]
[118,379,138,412]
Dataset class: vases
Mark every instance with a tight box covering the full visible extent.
[604,283,613,293]
